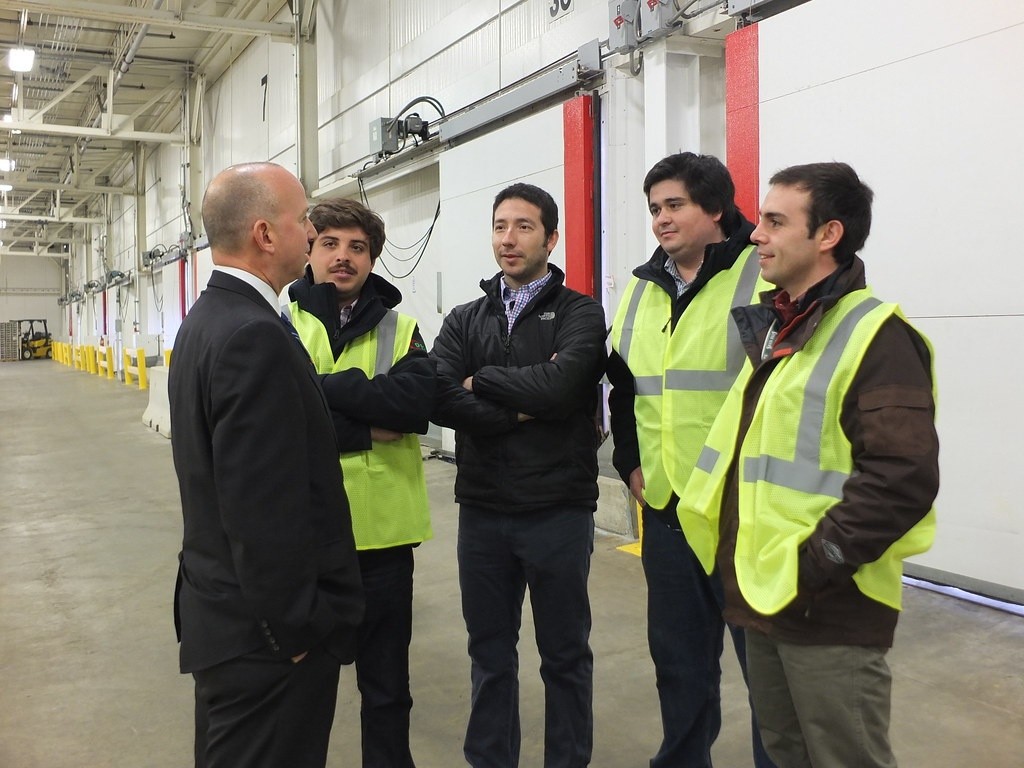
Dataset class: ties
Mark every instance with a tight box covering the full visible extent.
[280,309,311,360]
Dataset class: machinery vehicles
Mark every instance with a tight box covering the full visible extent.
[9,318,52,360]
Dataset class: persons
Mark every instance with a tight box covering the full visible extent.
[423,184,606,767]
[608,155,777,768]
[169,162,360,768]
[675,163,941,768]
[282,199,436,768]
